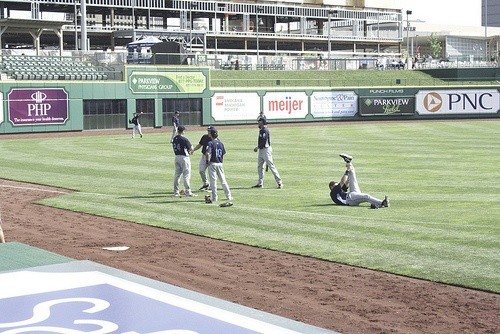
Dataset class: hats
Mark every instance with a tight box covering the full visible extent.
[177,125,186,131]
[175,111,181,114]
[207,126,215,131]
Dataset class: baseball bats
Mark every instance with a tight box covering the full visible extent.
[265,163,269,172]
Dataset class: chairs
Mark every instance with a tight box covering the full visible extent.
[220,63,285,70]
[3,56,107,80]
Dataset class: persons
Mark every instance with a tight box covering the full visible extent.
[129,112,145,140]
[328,153,390,209]
[169,111,182,143]
[171,125,198,198]
[250,114,284,189]
[204,127,234,201]
[192,127,220,190]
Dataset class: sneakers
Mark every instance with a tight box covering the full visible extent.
[200,183,210,191]
[251,183,263,188]
[174,192,180,196]
[187,193,198,197]
[211,197,217,201]
[277,183,283,189]
[383,196,389,207]
[339,153,352,162]
